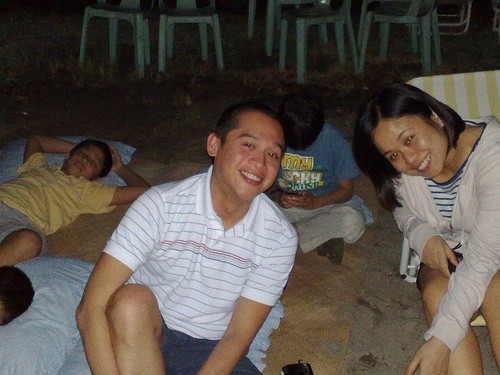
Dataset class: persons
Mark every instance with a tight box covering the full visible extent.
[273,82,367,264]
[73,102,299,375]
[0,131,151,326]
[349,81,500,375]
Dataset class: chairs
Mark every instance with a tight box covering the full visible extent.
[247,0,329,56]
[359,0,442,77]
[79,0,151,73]
[158,1,223,72]
[399,70,500,324]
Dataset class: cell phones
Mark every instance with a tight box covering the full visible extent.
[286,192,302,198]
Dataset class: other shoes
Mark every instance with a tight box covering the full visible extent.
[317,238,343,265]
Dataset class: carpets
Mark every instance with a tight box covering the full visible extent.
[45,161,381,375]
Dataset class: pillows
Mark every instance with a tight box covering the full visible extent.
[1,134,136,188]
[0,256,96,374]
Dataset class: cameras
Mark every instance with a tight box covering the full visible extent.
[281,362,313,375]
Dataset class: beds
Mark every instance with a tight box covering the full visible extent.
[280,1,358,82]
